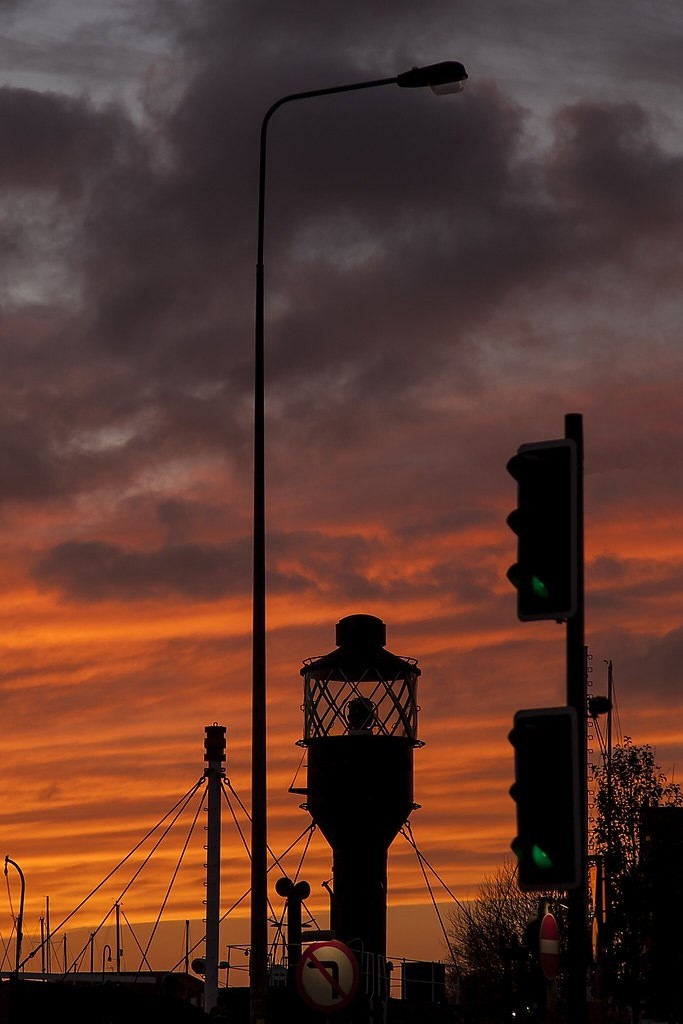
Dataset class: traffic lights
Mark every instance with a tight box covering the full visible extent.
[508,707,578,891]
[505,438,578,621]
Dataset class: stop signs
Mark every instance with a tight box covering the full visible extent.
[540,912,561,980]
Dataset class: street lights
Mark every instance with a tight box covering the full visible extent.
[252,63,468,1024]
